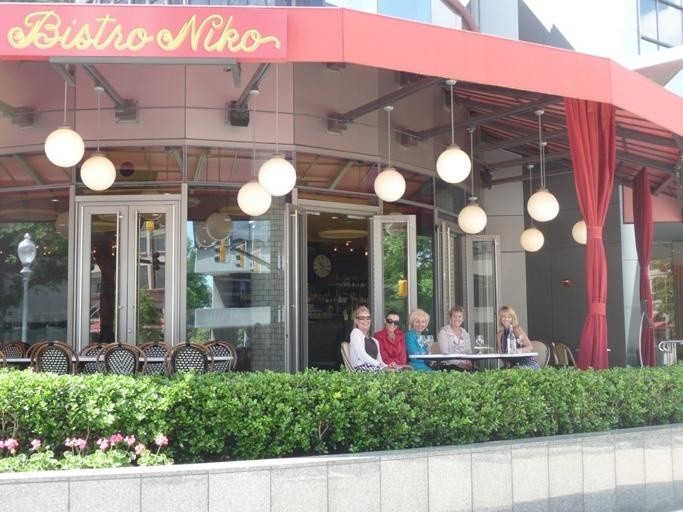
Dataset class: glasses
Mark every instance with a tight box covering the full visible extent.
[356,316,371,320]
[386,319,399,325]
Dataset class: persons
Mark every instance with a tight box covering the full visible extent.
[348,305,400,374]
[496,305,542,370]
[373,311,407,370]
[405,307,462,372]
[436,304,473,371]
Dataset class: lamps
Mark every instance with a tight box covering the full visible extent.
[206,209,234,241]
[56,210,69,240]
[43,65,85,168]
[237,86,273,217]
[258,64,297,197]
[373,79,588,253]
[193,221,216,247]
[80,84,117,191]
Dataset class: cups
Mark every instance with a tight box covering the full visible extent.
[418,334,434,354]
[476,335,484,353]
[516,336,526,353]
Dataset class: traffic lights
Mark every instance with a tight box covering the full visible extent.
[151,250,160,271]
[397,279,407,297]
[234,244,244,269]
[212,239,225,263]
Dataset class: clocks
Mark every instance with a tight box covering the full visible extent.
[313,254,332,278]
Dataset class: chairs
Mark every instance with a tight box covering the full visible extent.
[0,338,238,376]
[339,336,577,369]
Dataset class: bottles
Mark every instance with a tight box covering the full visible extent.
[506,324,517,353]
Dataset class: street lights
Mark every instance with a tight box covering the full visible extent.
[248,249,261,273]
[17,232,36,346]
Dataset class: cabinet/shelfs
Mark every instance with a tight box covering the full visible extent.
[308,276,368,319]
